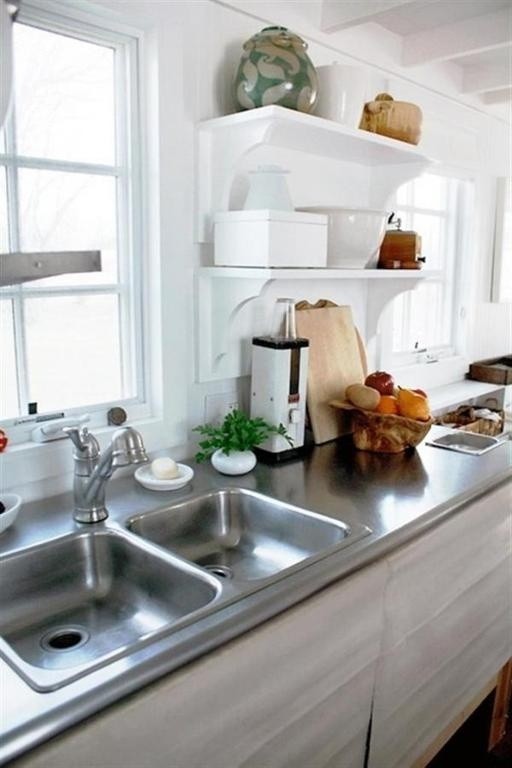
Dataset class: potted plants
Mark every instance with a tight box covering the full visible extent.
[189,408,296,476]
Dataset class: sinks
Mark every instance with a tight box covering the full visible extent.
[119,485,372,588]
[0,525,223,695]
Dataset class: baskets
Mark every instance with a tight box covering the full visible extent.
[432,398,505,436]
[359,92,424,145]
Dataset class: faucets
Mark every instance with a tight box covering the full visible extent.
[63,424,148,525]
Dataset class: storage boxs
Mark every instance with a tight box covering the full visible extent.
[215,210,329,268]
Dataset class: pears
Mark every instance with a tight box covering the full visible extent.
[396,385,429,421]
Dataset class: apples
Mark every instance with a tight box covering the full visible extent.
[364,370,393,395]
[410,389,427,397]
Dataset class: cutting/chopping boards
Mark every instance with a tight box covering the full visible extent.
[295,305,366,445]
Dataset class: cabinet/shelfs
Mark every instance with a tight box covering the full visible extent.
[194,104,429,383]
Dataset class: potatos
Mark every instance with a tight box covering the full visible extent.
[345,384,380,410]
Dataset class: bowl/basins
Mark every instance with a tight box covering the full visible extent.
[329,398,432,452]
[0,494,22,534]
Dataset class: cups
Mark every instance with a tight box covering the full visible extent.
[273,298,297,341]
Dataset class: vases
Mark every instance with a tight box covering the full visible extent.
[313,65,344,121]
